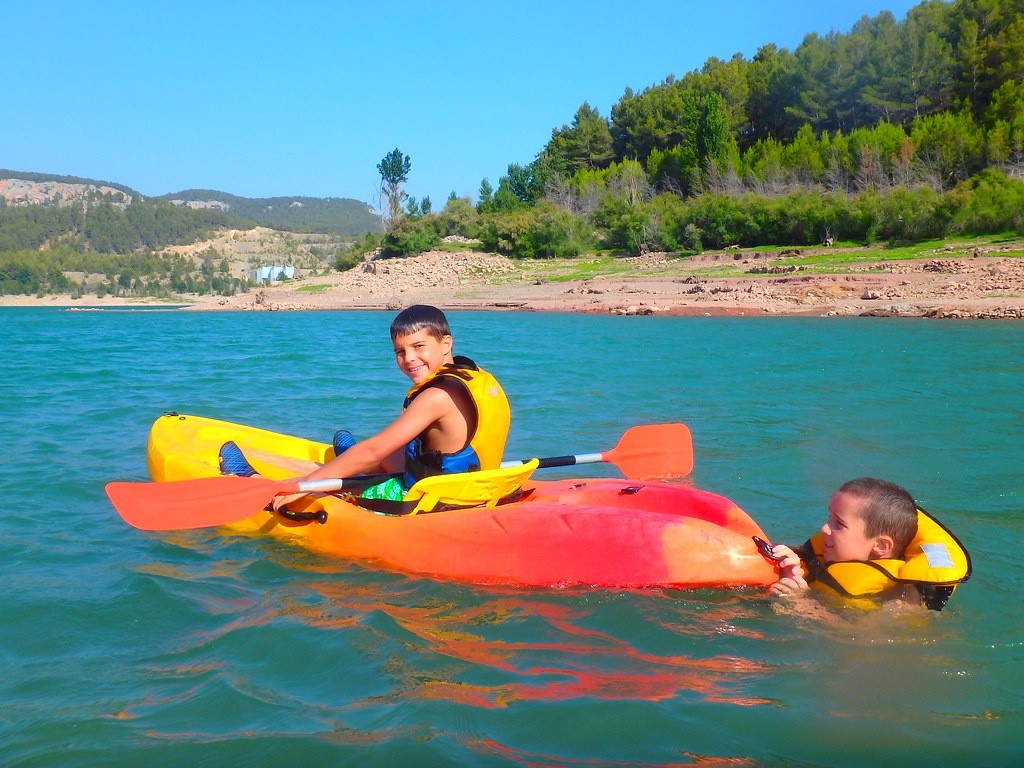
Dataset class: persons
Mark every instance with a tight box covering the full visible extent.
[768,477,918,597]
[218,304,512,516]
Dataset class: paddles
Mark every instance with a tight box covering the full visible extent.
[102,420,696,536]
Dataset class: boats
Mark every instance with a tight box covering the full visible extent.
[147,412,782,588]
